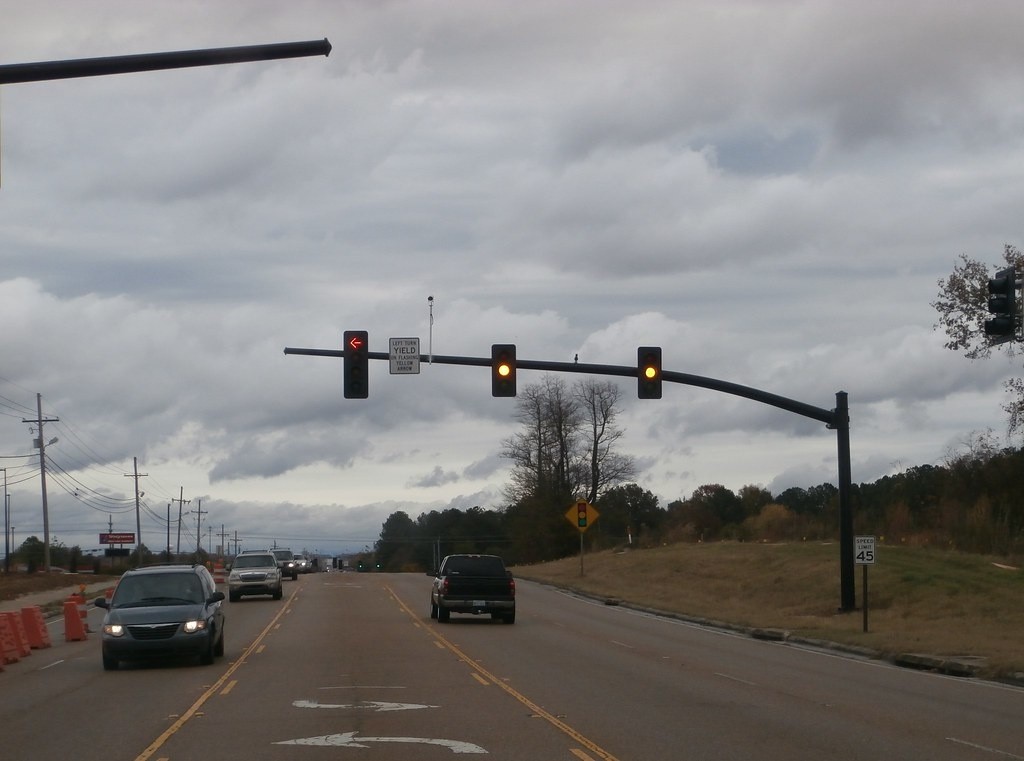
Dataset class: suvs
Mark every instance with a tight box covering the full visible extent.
[94,563,223,668]
[225,547,284,601]
[269,547,297,580]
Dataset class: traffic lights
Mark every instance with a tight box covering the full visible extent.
[637,346,663,399]
[343,330,369,399]
[492,344,515,397]
[578,503,587,527]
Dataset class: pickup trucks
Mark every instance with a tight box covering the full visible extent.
[427,554,516,624]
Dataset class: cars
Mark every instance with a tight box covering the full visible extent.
[293,555,307,573]
